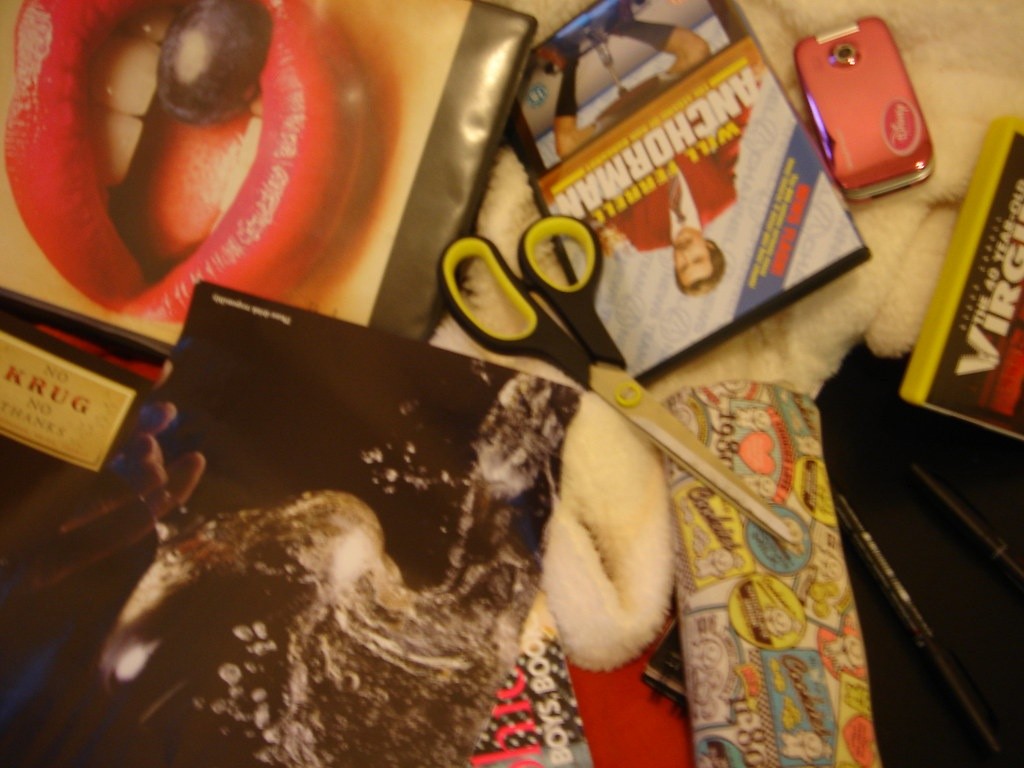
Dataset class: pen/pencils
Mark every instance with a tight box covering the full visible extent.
[831,487,1003,754]
[909,462,1024,590]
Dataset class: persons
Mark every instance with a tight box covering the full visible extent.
[0,403,206,611]
[539,0,767,297]
[0,0,482,347]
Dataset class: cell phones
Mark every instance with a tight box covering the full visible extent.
[794,16,934,201]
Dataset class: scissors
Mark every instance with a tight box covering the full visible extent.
[435,213,799,548]
